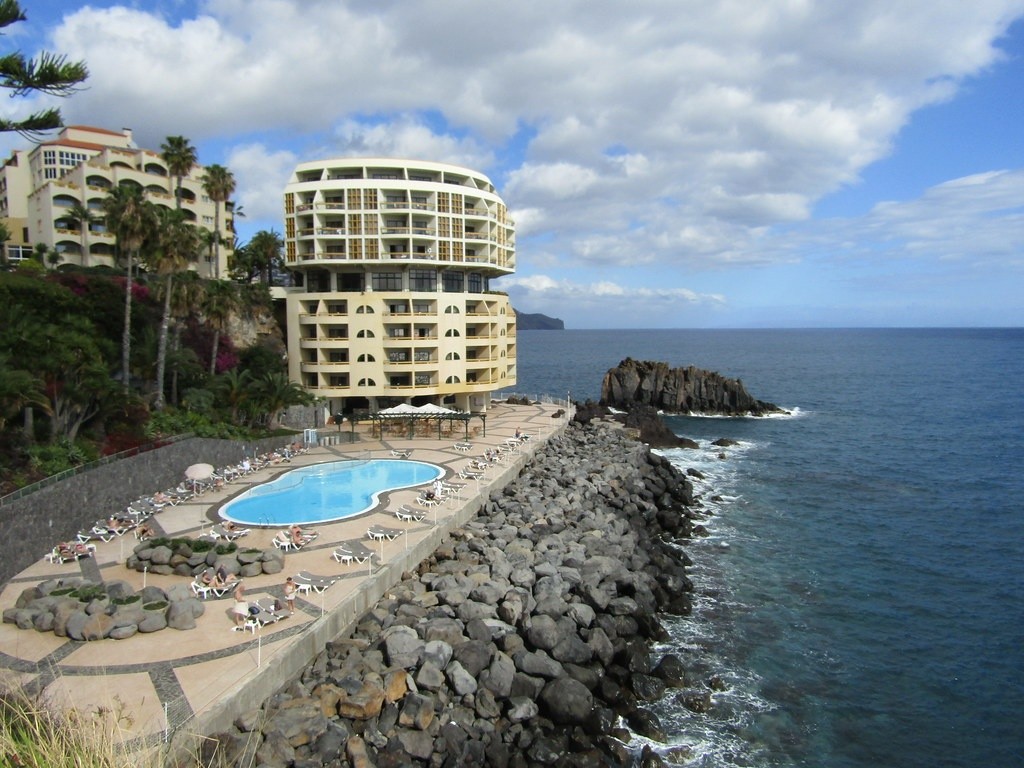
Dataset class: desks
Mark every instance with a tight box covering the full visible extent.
[425,501,434,509]
[340,555,353,566]
[298,584,312,596]
[401,515,412,523]
[373,534,385,542]
[279,542,292,552]
[492,457,498,463]
[44,553,57,563]
[209,533,220,539]
[197,588,212,600]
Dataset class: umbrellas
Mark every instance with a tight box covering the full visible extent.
[184,463,214,480]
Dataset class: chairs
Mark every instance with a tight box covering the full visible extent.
[43,431,530,634]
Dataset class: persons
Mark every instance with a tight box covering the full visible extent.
[139,522,153,537]
[226,522,243,531]
[154,490,175,504]
[109,517,119,528]
[284,577,295,615]
[425,478,442,501]
[202,564,237,587]
[516,425,521,437]
[290,524,306,546]
[292,443,307,450]
[235,585,245,625]
[267,452,279,460]
[56,544,88,555]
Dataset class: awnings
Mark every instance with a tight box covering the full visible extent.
[378,403,460,416]
